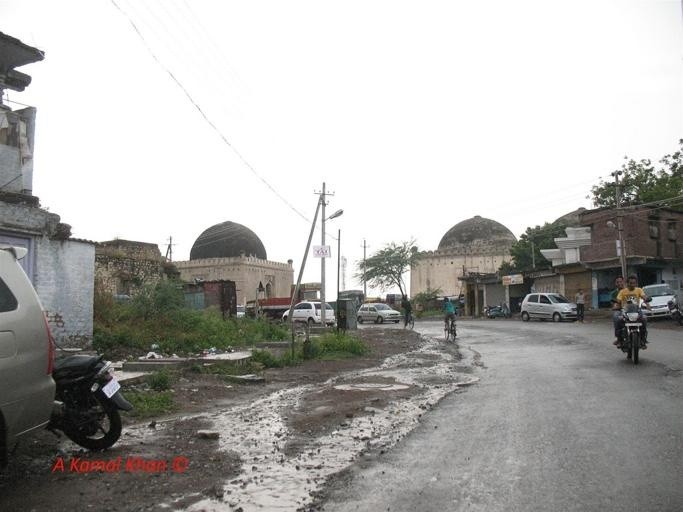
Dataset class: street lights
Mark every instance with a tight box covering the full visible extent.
[320,183,344,329]
[361,254,372,297]
[604,221,627,285]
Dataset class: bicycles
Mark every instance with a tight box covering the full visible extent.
[402,310,415,330]
[444,318,457,342]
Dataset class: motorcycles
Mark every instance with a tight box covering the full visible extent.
[666,294,683,327]
[43,347,133,452]
[610,295,652,365]
[486,301,513,319]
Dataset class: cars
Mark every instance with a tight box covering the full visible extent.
[520,292,582,322]
[638,282,677,321]
[281,301,336,326]
[356,302,402,325]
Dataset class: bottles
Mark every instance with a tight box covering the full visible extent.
[208,346,217,353]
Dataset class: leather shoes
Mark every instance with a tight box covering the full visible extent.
[614,340,622,348]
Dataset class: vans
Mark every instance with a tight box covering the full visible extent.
[235,304,245,318]
[0,244,57,469]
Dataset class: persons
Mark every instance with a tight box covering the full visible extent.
[575,288,585,324]
[459,293,464,316]
[612,276,625,345]
[401,296,412,326]
[441,297,457,330]
[617,275,651,348]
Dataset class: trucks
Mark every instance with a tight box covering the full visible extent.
[245,283,321,320]
